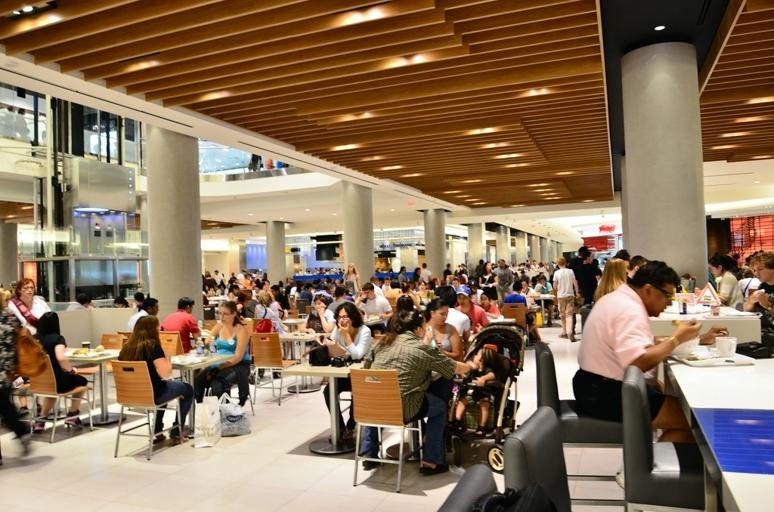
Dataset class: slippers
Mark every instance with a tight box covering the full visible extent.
[362,456,380,467]
[153,436,166,444]
[170,437,188,446]
[418,463,450,475]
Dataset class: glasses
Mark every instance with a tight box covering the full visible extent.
[654,286,674,301]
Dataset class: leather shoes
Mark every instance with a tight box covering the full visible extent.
[569,333,576,342]
[560,333,568,339]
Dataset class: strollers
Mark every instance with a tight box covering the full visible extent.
[447,323,530,474]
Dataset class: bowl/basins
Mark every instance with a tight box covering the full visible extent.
[660,336,699,357]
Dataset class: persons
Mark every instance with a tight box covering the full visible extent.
[0,278,88,468]
[114,297,130,308]
[248,153,288,169]
[120,246,773,475]
[68,295,95,311]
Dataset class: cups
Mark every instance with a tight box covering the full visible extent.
[81,341,91,353]
[716,336,738,358]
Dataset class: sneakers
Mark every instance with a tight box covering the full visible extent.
[32,420,45,432]
[344,430,353,439]
[65,409,83,428]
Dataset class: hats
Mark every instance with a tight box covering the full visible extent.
[455,284,472,298]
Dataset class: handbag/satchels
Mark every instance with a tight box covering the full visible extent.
[306,311,325,346]
[193,386,221,449]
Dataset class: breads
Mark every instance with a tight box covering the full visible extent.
[72,348,89,354]
[94,345,104,352]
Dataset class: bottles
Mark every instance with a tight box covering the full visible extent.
[676,284,720,316]
[208,336,217,359]
[196,336,205,360]
